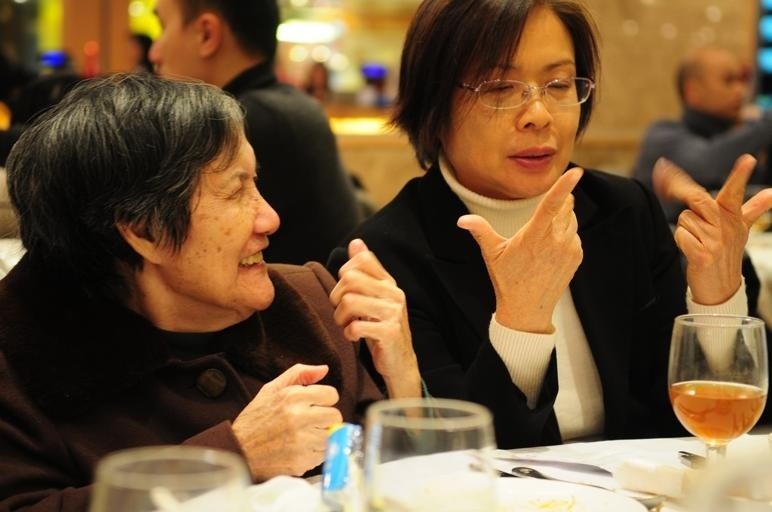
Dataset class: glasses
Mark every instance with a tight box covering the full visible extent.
[459,76,597,111]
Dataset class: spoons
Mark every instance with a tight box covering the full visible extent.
[510,464,669,508]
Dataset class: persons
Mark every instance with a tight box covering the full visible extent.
[354,60,397,106]
[0,71,456,512]
[145,0,355,264]
[630,45,772,220]
[307,61,335,103]
[123,34,158,78]
[329,1,772,452]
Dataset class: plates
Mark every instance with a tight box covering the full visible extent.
[493,476,648,512]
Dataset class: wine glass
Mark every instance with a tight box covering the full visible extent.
[668,313,768,468]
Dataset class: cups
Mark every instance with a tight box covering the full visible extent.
[92,445,253,512]
[366,398,498,512]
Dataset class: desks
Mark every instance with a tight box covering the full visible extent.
[740,230,772,321]
